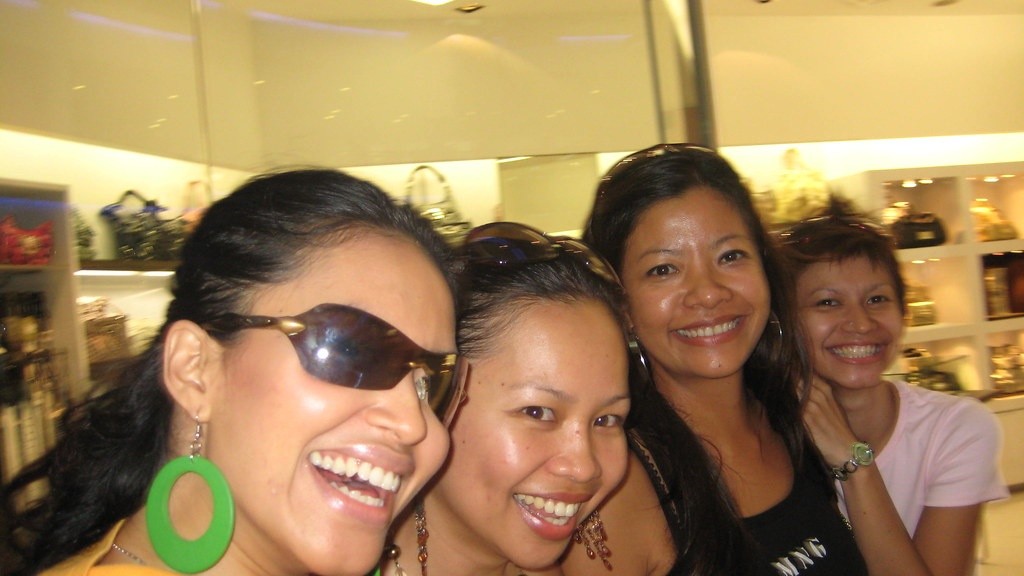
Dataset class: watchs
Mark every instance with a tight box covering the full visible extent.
[830,441,874,480]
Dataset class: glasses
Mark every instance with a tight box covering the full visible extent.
[778,215,894,247]
[461,221,627,299]
[195,302,470,431]
[588,142,717,244]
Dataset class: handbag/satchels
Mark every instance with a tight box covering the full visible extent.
[78,296,161,379]
[903,284,938,328]
[981,252,1024,319]
[100,176,217,261]
[989,344,1024,394]
[878,199,947,249]
[971,197,1016,243]
[406,165,472,246]
[1,215,57,265]
[900,347,961,397]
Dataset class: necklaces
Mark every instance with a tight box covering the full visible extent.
[111,543,141,564]
[415,495,429,576]
[385,535,403,576]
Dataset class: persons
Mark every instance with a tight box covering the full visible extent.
[0,166,471,576]
[775,195,1010,576]
[564,143,874,576]
[369,220,647,576]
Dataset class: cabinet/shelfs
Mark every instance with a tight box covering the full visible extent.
[0,179,182,522]
[838,160,1024,486]
